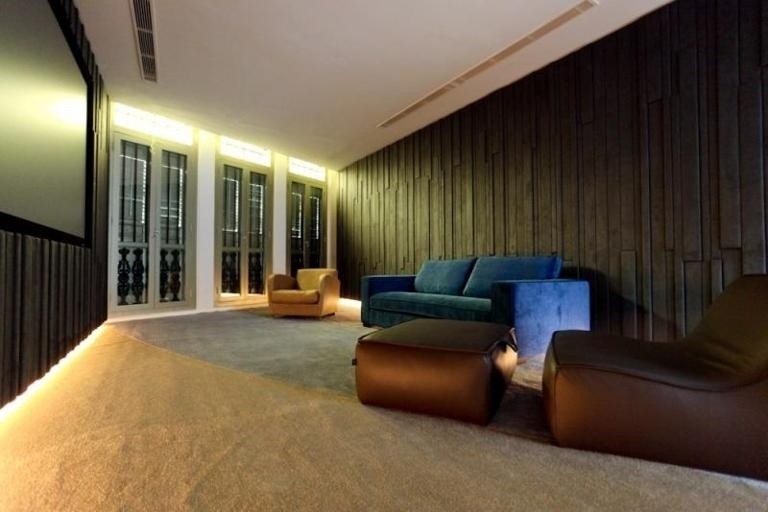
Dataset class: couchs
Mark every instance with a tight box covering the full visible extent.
[361,256,591,355]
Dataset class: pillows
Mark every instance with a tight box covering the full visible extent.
[414,255,477,294]
[463,254,562,298]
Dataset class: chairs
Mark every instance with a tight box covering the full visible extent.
[267,268,341,319]
[542,273,768,482]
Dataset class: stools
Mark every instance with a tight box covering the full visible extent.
[356,318,519,426]
